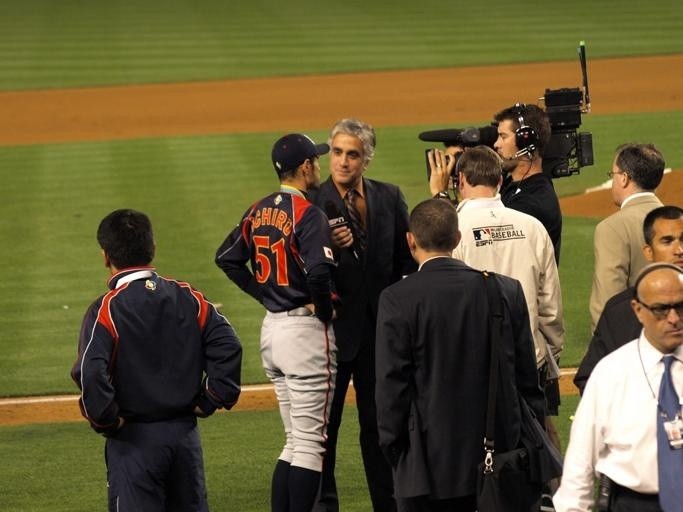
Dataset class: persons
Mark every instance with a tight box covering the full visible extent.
[214,132,340,510]
[425,100,564,272]
[547,262,682,512]
[313,119,412,511]
[69,207,243,511]
[372,196,565,511]
[449,140,567,419]
[572,205,683,398]
[584,142,668,339]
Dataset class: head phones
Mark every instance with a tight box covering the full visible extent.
[515,103,537,152]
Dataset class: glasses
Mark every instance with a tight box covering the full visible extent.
[636,299,683,318]
[608,169,632,180]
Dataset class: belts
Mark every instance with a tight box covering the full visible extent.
[286,307,313,316]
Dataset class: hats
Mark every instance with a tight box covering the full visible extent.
[272,132,330,173]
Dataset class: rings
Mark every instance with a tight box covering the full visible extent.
[436,165,441,168]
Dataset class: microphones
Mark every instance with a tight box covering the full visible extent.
[509,147,529,160]
[324,201,361,265]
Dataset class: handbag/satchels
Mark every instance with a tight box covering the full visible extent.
[477,448,538,512]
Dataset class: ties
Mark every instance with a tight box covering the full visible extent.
[657,357,683,512]
[346,191,368,251]
